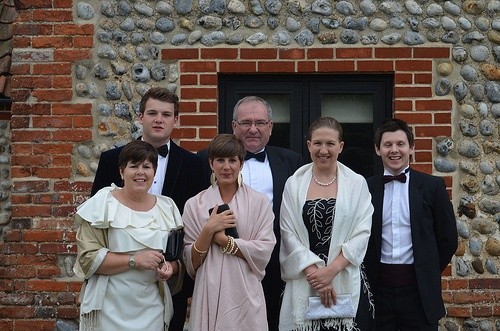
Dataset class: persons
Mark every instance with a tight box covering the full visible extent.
[196,96,304,331]
[90,87,202,331]
[367,118,458,331]
[72,141,184,331]
[182,133,277,331]
[279,117,375,331]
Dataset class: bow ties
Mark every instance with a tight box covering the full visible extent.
[380,166,410,183]
[156,144,168,158]
[244,150,266,162]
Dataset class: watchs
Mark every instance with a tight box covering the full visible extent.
[128,255,135,270]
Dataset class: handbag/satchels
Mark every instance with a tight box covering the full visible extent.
[305,294,356,319]
[209,203,239,238]
[165,226,185,261]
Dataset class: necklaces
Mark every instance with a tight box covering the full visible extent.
[313,173,336,186]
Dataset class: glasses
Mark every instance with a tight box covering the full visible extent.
[236,119,270,127]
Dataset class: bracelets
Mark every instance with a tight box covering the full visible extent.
[157,254,168,273]
[193,242,208,253]
[220,234,239,256]
